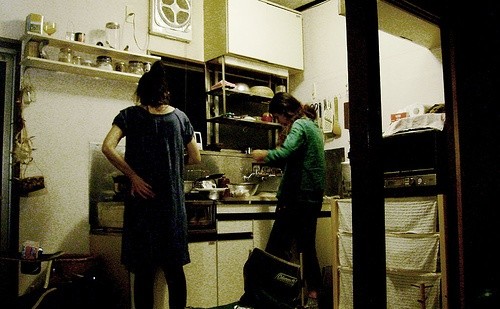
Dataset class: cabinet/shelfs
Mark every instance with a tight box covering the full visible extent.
[19,31,162,84]
[331,195,448,309]
[205,57,289,153]
[204,0,303,75]
[90,200,331,309]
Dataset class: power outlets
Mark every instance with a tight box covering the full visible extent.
[126,5,136,22]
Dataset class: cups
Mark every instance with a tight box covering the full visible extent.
[65,32,83,42]
[106,22,120,50]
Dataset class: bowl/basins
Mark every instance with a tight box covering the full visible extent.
[234,83,249,93]
[226,184,260,196]
[249,86,274,97]
[184,181,194,193]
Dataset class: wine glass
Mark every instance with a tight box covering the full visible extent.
[43,20,57,36]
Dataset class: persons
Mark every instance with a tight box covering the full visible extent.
[251,92,327,309]
[102,59,201,309]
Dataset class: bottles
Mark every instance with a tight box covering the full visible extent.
[23,88,32,105]
[28,40,152,75]
